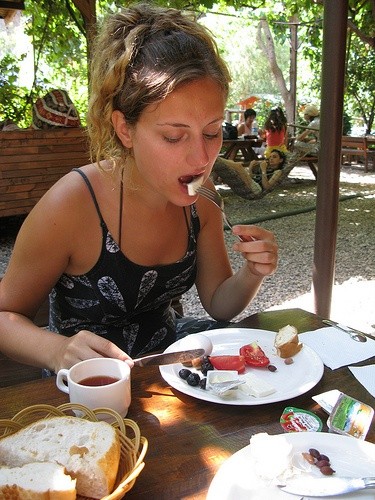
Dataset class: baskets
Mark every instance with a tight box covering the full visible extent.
[0,402,148,500]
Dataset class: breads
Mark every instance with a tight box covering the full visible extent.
[275,325,303,358]
[0,416,121,500]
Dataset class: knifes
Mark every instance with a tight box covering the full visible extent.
[133,349,205,367]
[278,475,375,498]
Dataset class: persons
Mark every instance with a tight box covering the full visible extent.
[0,3,278,376]
[237,107,288,158]
[219,149,287,194]
[293,106,321,152]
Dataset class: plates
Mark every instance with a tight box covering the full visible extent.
[205,432,375,500]
[158,327,324,406]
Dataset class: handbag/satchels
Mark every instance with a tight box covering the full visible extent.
[31,89,82,130]
[222,122,239,140]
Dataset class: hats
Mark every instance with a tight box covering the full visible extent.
[303,106,318,117]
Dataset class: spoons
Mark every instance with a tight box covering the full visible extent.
[321,319,367,342]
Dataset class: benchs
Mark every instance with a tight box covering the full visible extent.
[232,156,318,178]
[342,136,375,174]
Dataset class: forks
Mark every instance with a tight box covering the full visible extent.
[195,184,248,243]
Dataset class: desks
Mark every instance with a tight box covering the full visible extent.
[0,309,375,500]
[213,140,263,185]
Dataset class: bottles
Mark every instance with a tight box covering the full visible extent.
[251,118,258,137]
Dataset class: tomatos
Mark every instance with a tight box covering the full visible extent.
[240,342,270,367]
[208,353,245,372]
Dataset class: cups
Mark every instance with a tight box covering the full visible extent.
[56,357,132,424]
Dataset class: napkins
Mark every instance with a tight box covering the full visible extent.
[347,365,375,398]
[298,324,375,370]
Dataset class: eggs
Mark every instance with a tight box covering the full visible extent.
[178,333,212,362]
